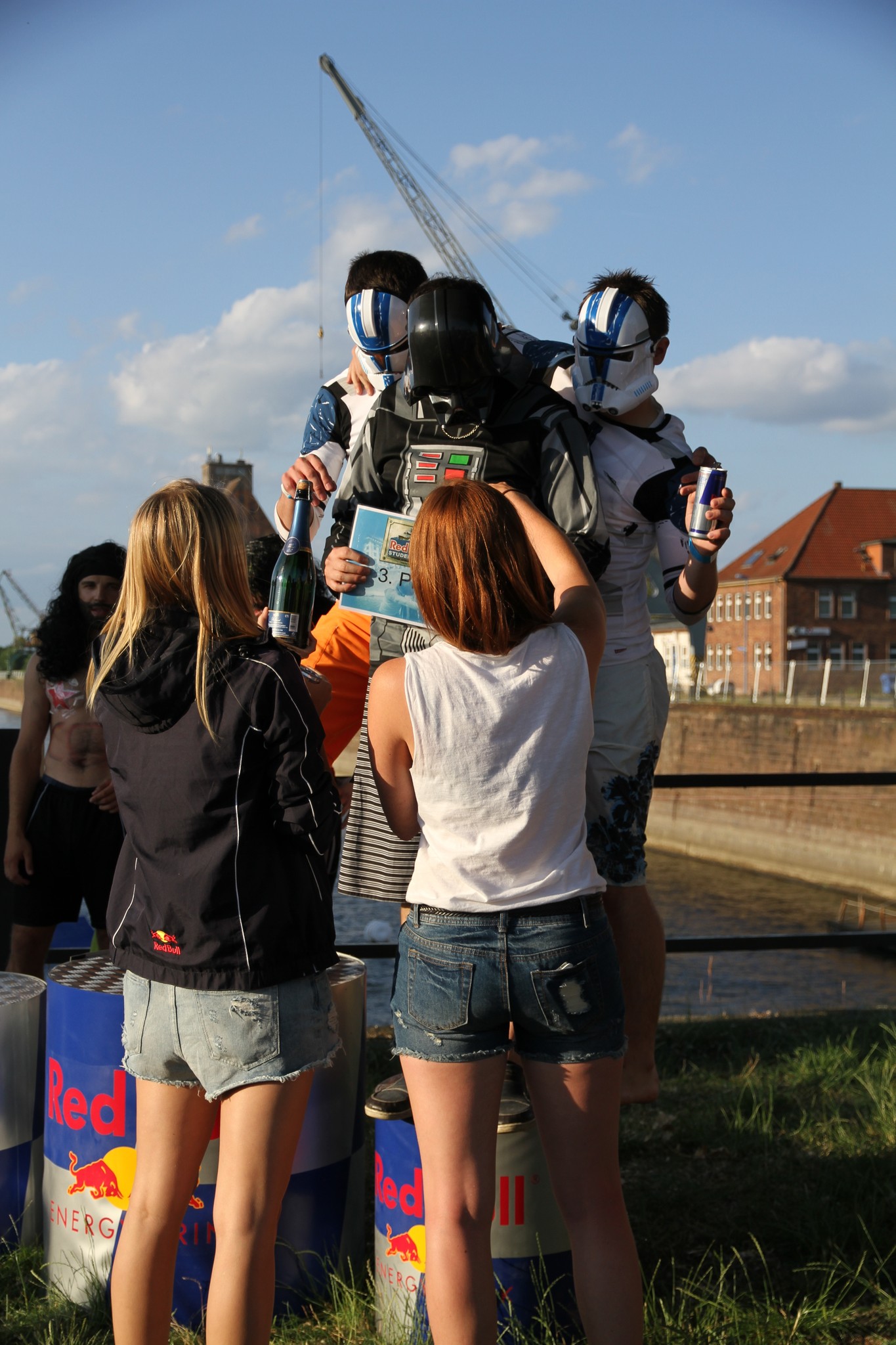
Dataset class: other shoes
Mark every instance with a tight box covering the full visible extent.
[496,1061,535,1135]
[364,1073,413,1120]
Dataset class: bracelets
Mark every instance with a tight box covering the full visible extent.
[687,538,718,565]
[281,484,292,500]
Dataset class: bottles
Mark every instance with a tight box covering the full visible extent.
[267,479,316,666]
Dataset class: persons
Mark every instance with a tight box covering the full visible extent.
[221,249,734,1101]
[84,477,338,1345]
[336,484,643,1345]
[4,539,127,973]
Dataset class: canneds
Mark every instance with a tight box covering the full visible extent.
[688,461,728,541]
[299,664,322,684]
